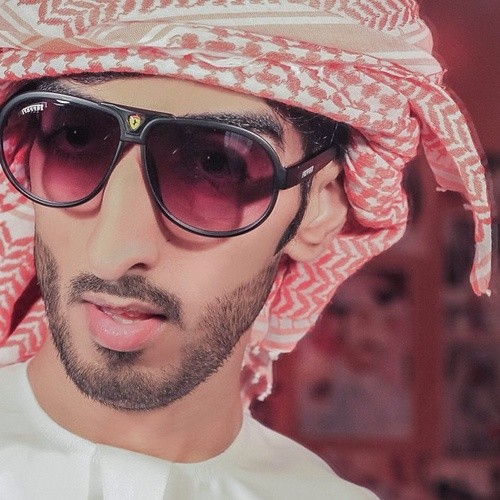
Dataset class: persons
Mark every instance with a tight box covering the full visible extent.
[1,1,493,500]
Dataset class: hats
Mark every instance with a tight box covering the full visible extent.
[2,0,491,407]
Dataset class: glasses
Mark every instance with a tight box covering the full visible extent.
[0,90,340,238]
